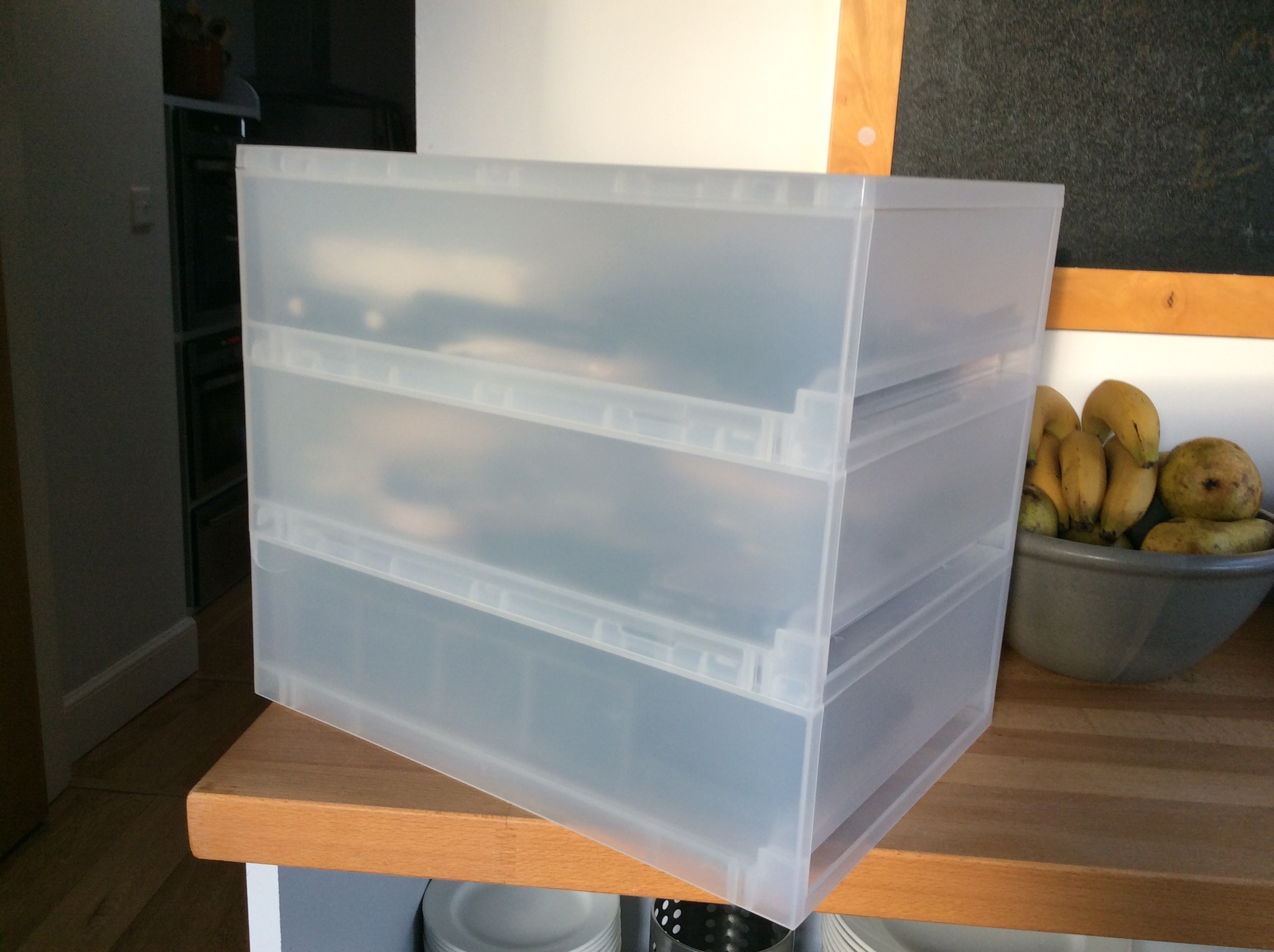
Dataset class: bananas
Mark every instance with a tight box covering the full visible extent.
[1026,379,1162,544]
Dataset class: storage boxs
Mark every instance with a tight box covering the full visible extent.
[231,139,1074,932]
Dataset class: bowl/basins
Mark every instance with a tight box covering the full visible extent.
[1005,507,1273,685]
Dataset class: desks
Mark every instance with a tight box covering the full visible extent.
[193,595,1274,952]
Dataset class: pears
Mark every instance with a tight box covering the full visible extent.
[1157,435,1262,521]
[1019,481,1134,555]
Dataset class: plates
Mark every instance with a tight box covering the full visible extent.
[420,879,624,950]
[820,913,1137,952]
